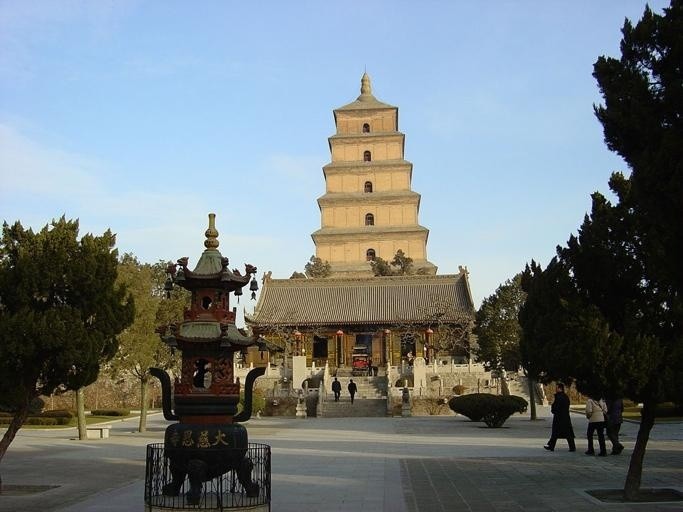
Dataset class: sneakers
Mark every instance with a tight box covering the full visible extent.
[543,444,624,457]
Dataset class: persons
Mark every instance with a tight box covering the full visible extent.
[400,351,415,365]
[605,399,624,456]
[584,394,608,458]
[367,359,378,376]
[348,379,357,406]
[544,383,576,452]
[332,378,341,403]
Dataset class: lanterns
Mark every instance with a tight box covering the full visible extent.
[384,329,391,336]
[296,332,301,338]
[335,330,344,337]
[426,328,434,336]
